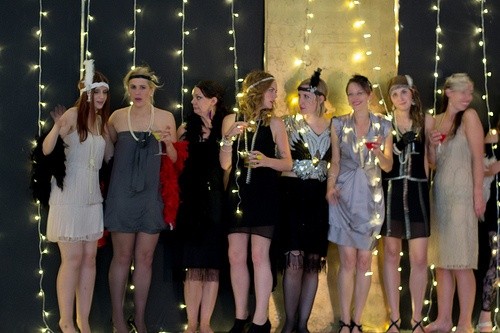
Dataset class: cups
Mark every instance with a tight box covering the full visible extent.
[244,153,257,168]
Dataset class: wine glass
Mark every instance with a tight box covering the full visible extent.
[404,127,421,154]
[436,128,446,152]
[363,137,378,165]
[149,127,167,156]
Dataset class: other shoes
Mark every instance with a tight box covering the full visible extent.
[127,318,138,333]
[386,318,400,333]
[411,317,426,333]
[246,320,272,333]
[75,321,81,333]
[112,323,117,333]
[350,320,362,333]
[58,324,63,333]
[228,313,252,333]
[338,320,352,333]
[478,318,493,333]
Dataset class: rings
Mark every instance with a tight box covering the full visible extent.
[256,155,262,160]
[168,131,172,136]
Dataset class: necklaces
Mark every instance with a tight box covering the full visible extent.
[86,116,100,195]
[243,112,262,185]
[127,104,154,142]
[394,113,413,164]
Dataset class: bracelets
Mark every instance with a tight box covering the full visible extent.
[220,136,233,153]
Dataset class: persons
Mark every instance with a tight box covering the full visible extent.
[174,81,229,333]
[326,75,394,333]
[219,70,293,333]
[423,73,486,333]
[380,74,435,333]
[276,68,337,333]
[105,67,177,333]
[476,120,500,333]
[42,71,109,333]
